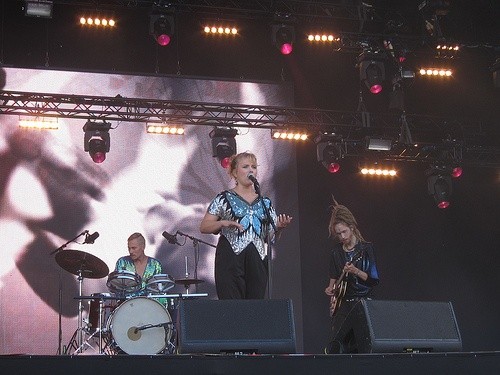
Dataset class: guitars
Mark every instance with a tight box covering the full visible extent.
[330,252,363,318]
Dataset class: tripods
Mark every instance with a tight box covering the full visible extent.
[64,264,131,356]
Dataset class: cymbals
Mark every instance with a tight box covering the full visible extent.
[175,277,205,285]
[88,291,126,338]
[55,249,108,279]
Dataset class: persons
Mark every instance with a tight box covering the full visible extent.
[324,205,379,353]
[115,232,166,305]
[200,152,293,299]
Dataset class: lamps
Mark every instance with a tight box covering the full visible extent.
[82,121,110,163]
[446,165,462,177]
[146,121,185,136]
[209,124,238,168]
[17,114,58,130]
[359,157,396,176]
[77,10,460,93]
[428,177,453,209]
[269,128,308,141]
[320,148,340,173]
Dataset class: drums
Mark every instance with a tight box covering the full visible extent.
[106,270,142,293]
[145,274,175,292]
[148,294,173,313]
[106,296,174,355]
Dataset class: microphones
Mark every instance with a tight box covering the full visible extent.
[134,328,139,334]
[162,230,180,246]
[82,232,99,244]
[247,173,260,186]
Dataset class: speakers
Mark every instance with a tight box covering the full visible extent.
[175,298,297,354]
[330,301,463,354]
